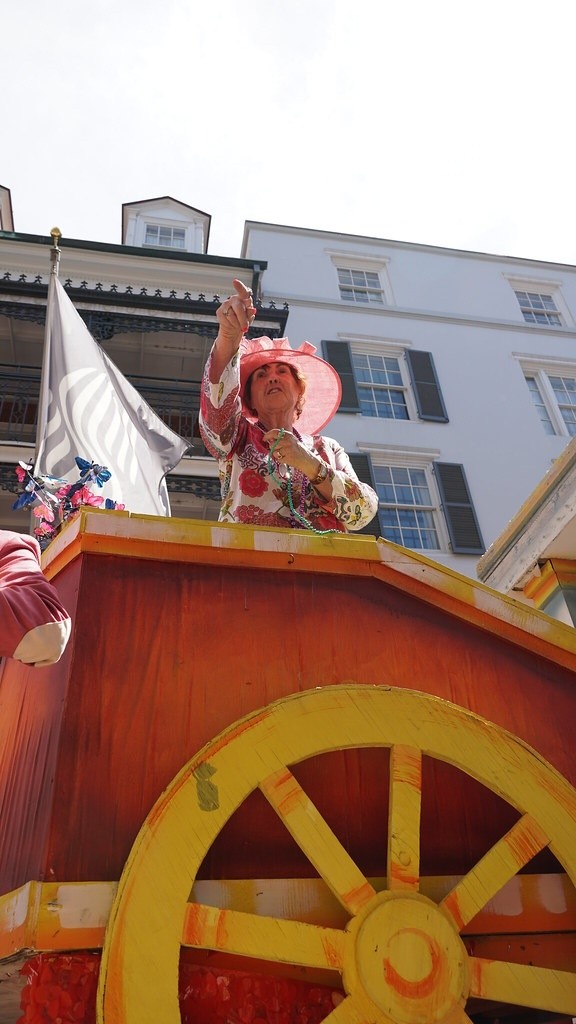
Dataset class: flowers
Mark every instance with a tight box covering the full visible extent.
[7,457,125,538]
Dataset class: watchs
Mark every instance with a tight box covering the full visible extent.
[310,460,327,485]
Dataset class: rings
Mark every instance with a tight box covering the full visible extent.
[277,450,283,460]
[225,306,232,316]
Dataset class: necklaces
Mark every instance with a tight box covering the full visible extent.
[256,420,343,535]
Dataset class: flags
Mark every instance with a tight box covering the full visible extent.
[33,277,194,517]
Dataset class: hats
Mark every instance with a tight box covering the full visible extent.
[238,334,343,436]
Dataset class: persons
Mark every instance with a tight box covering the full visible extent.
[0,529,72,666]
[197,278,378,534]
